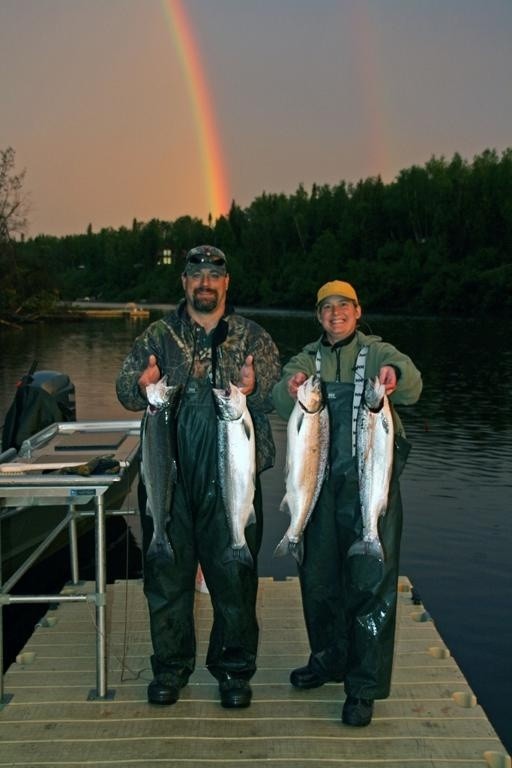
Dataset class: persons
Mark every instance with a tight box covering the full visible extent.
[111,244,284,708]
[269,278,428,728]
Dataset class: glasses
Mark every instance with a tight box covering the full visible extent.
[186,253,228,267]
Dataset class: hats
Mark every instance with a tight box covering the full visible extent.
[314,279,359,309]
[183,244,231,278]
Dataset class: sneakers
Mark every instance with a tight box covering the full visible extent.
[219,689,252,709]
[145,679,180,707]
[289,664,319,690]
[340,694,376,728]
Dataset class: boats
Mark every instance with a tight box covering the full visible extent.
[0,414,149,579]
